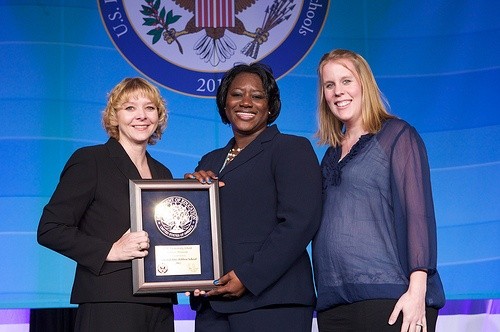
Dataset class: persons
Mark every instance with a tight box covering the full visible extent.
[183,63,325,332]
[311,48,445,332]
[36,75,206,332]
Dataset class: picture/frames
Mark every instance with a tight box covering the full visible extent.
[129,178,225,294]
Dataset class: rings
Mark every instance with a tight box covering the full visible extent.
[137,243,142,251]
[416,323,423,327]
